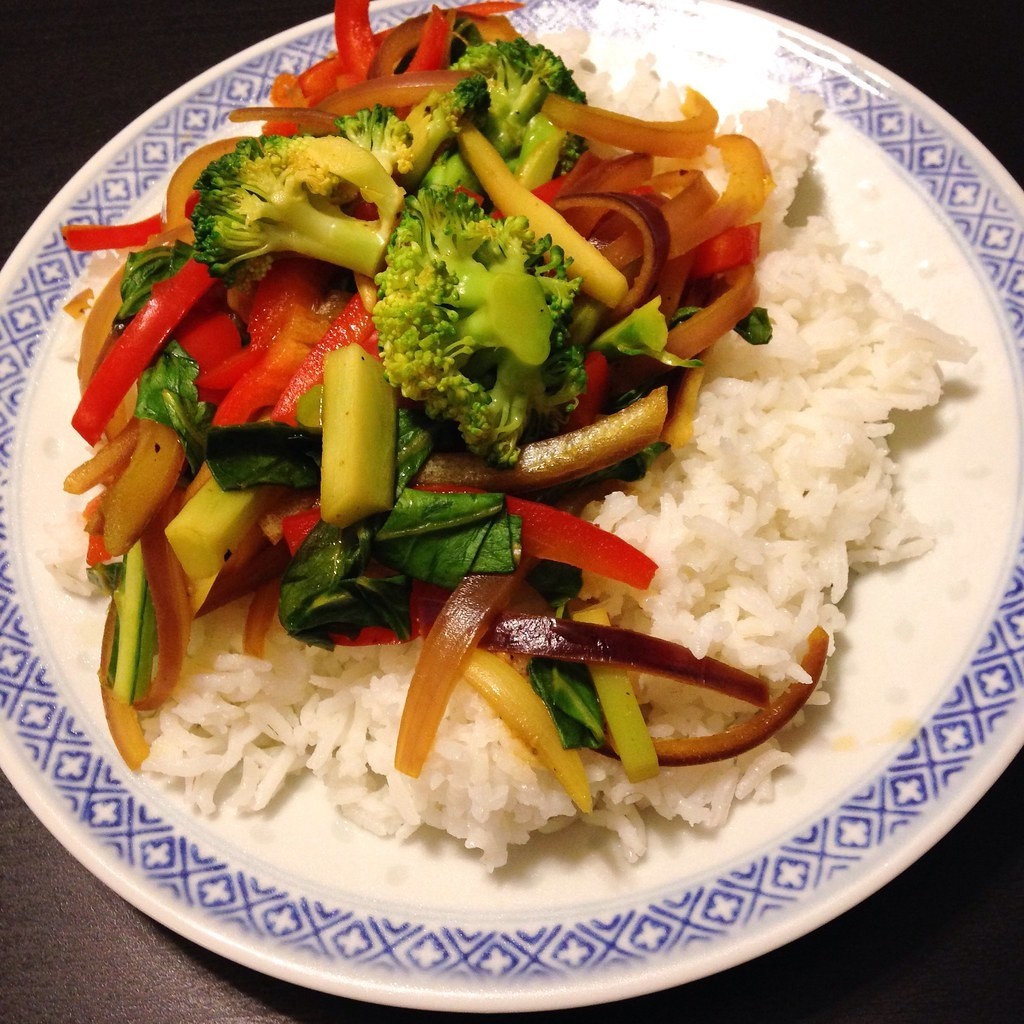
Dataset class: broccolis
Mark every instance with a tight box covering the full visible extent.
[117,37,666,465]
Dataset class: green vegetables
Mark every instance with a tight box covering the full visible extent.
[85,240,605,758]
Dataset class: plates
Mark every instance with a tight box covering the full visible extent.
[0,0,1024,1013]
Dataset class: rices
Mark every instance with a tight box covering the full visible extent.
[143,48,975,875]
